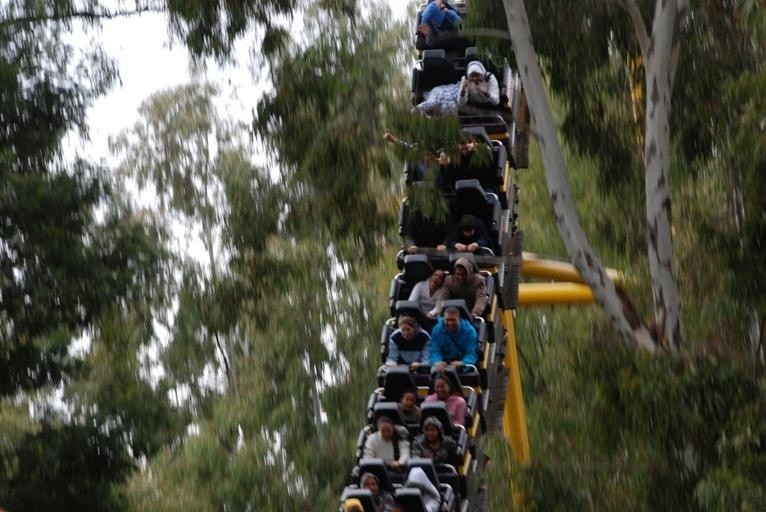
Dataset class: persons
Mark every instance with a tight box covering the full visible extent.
[336,0,512,511]
